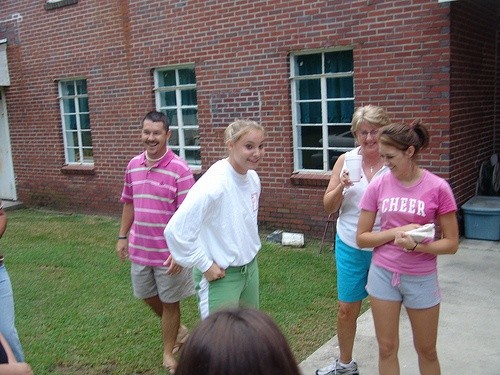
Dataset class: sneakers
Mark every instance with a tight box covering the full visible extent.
[316,359,359,375]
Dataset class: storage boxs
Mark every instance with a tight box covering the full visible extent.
[461,196,500,240]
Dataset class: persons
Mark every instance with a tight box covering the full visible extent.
[0,198,33,375]
[116,111,197,375]
[163,119,267,319]
[175,307,300,375]
[314,103,392,375]
[356,119,460,375]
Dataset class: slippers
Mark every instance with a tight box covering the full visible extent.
[162,363,177,375]
[171,335,190,357]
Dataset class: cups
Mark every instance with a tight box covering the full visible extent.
[344,155,363,182]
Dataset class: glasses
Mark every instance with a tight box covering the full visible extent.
[356,131,378,136]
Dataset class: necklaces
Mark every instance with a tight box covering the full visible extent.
[363,157,386,173]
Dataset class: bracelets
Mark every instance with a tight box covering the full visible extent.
[408,241,419,253]
[118,236,128,241]
